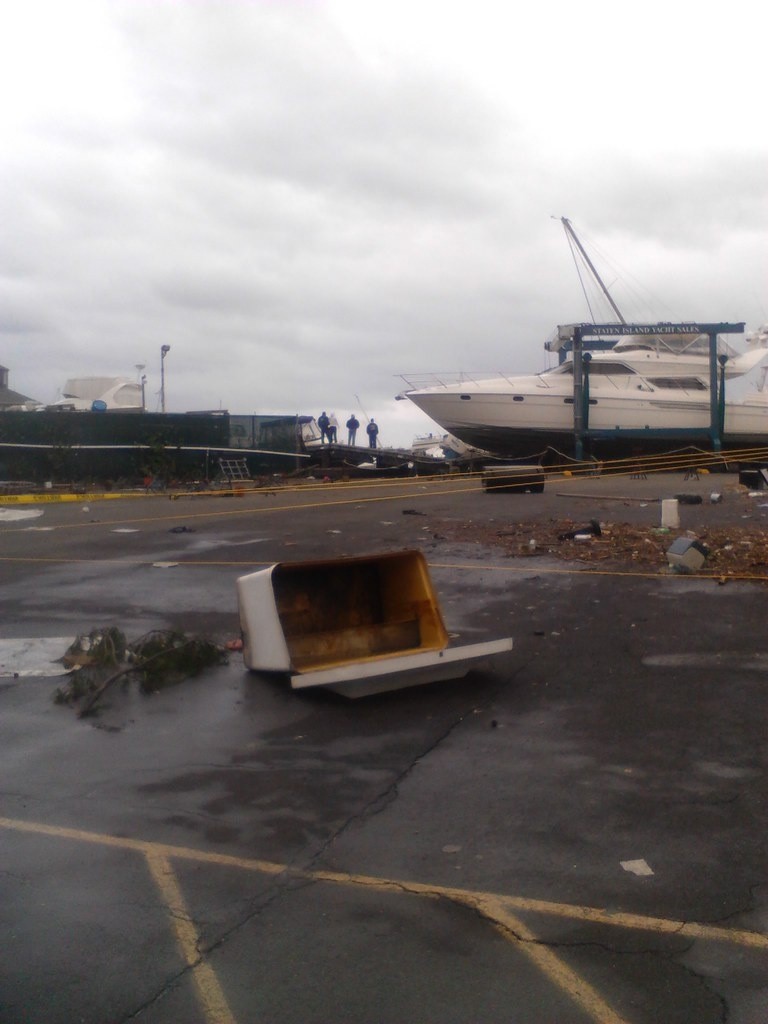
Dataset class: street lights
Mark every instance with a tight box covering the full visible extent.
[160,343,170,414]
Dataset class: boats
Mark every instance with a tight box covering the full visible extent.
[306,431,468,476]
[393,215,768,474]
[0,366,327,485]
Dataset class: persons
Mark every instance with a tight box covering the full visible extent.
[366,418,378,449]
[319,410,340,444]
[346,414,359,446]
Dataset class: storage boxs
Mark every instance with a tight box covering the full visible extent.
[234,550,515,701]
